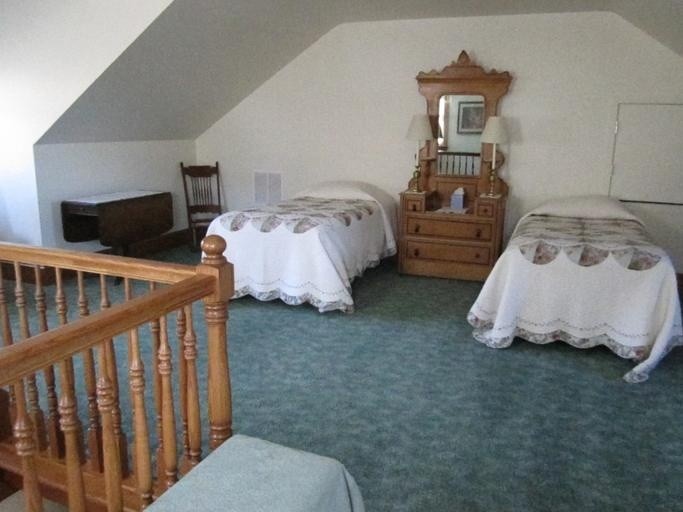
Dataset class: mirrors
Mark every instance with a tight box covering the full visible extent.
[408,49,514,190]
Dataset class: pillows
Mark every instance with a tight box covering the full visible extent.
[294,182,386,200]
[525,194,642,224]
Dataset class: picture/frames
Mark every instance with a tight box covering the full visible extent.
[457,100,483,133]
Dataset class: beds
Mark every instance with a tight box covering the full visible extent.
[461,197,683,383]
[203,183,399,312]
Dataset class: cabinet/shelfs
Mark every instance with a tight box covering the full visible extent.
[398,189,510,284]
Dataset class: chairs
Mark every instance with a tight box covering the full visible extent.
[181,161,223,251]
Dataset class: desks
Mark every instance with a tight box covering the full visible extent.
[140,430,367,511]
[60,189,174,285]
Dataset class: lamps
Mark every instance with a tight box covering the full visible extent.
[405,115,434,196]
[481,114,509,199]
